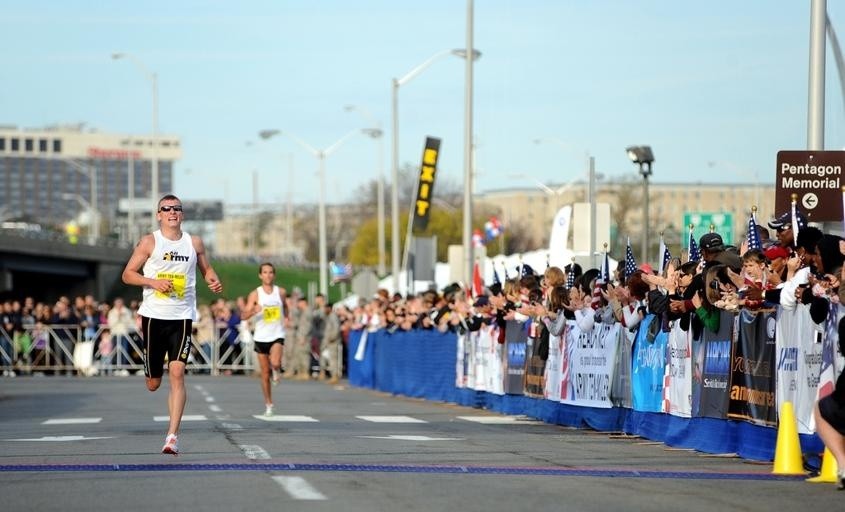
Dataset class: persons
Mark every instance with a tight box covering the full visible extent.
[795,270,845,494]
[353,211,845,332]
[243,264,288,417]
[1,292,353,383]
[121,194,223,455]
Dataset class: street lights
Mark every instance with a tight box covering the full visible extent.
[106,48,159,232]
[387,47,484,296]
[257,122,385,301]
[58,191,101,241]
[502,168,606,210]
[44,154,100,245]
[625,143,657,262]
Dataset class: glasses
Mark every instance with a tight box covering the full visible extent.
[680,274,688,278]
[777,225,791,231]
[160,205,182,212]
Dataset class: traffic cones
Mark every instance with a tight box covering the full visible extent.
[804,445,839,485]
[770,399,813,478]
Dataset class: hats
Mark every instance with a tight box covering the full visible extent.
[700,233,723,249]
[764,246,787,259]
[768,211,806,230]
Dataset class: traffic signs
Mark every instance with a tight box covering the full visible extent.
[773,148,845,224]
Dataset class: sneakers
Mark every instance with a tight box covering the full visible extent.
[161,433,179,454]
[265,403,273,417]
[272,369,280,385]
